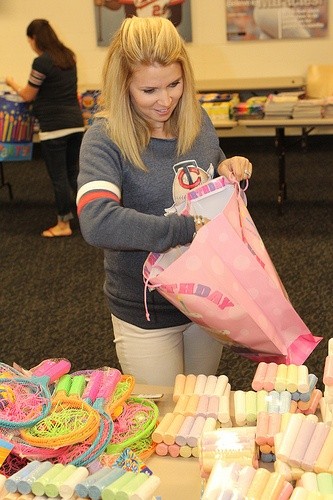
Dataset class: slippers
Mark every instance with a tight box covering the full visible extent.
[41,227,72,238]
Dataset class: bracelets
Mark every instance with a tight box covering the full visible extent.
[16,88,23,95]
[193,215,205,242]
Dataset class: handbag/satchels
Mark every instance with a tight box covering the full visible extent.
[141,173,322,366]
[0,81,34,163]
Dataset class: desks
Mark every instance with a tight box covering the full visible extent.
[238,116,333,216]
[193,76,314,152]
[130,384,323,500]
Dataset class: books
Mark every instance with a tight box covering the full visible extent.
[198,93,333,124]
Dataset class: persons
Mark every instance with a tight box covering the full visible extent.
[6,19,87,237]
[76,15,253,387]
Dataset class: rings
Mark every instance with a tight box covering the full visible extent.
[244,169,251,175]
